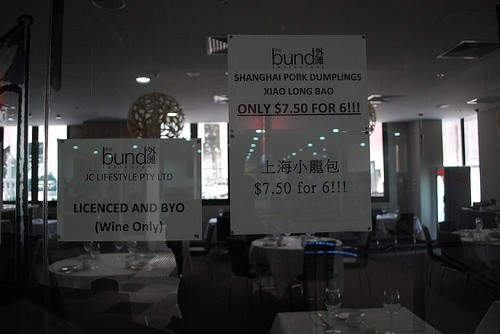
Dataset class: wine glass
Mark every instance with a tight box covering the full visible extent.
[382,288,401,334]
[322,287,343,334]
[83,240,137,273]
[271,219,315,246]
[474,216,500,235]
[380,205,400,217]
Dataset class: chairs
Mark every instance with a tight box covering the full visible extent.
[18,210,458,334]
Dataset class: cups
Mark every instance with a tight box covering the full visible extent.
[79,254,92,270]
[124,255,137,270]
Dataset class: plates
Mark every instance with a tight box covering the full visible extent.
[335,309,366,319]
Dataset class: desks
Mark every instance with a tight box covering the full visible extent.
[247,235,343,299]
[50,251,177,294]
[376,213,422,246]
[462,202,500,224]
[452,227,500,269]
[270,306,442,334]
[31,218,57,240]
